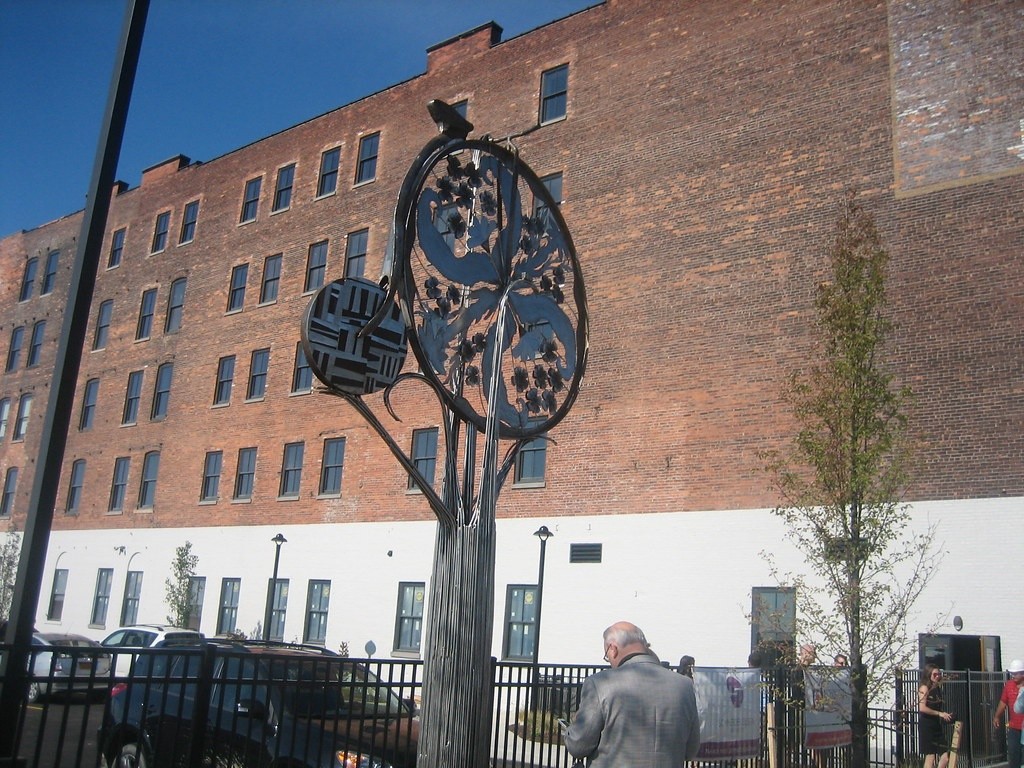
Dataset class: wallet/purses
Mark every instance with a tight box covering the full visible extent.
[941,714,958,724]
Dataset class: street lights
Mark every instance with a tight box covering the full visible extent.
[264,533,288,642]
[530,526,553,714]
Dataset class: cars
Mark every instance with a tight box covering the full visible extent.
[0,633,113,704]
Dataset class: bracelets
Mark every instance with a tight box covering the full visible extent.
[937,711,940,717]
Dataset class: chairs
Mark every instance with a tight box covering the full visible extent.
[292,670,336,713]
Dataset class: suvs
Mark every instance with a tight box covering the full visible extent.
[100,623,205,677]
[95,638,420,768]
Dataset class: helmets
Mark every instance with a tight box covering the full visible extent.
[1008,659,1024,672]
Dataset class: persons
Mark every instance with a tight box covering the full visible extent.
[833,654,856,768]
[992,658,1024,768]
[1013,686,1024,745]
[916,662,952,768]
[676,655,696,678]
[563,621,702,768]
[799,643,826,768]
[747,652,761,669]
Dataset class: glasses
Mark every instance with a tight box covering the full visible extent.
[604,644,613,662]
[933,673,941,676]
